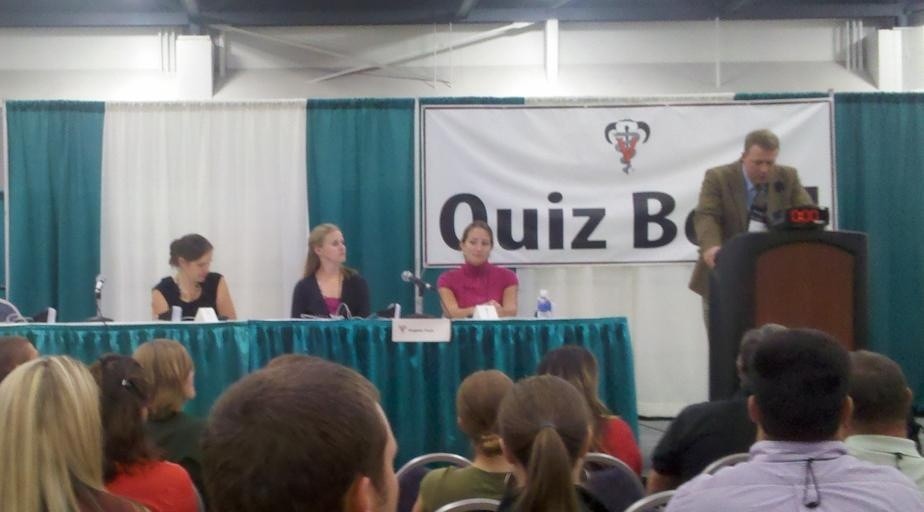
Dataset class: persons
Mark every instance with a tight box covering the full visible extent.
[414,346,643,511]
[291,221,373,320]
[2,335,398,512]
[437,219,519,320]
[150,234,237,321]
[649,322,923,512]
[688,127,818,402]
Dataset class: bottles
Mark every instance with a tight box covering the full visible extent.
[537,290,553,317]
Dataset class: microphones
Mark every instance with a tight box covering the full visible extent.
[94,274,105,298]
[400,270,435,294]
[774,179,784,194]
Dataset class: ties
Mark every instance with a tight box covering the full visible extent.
[748,184,768,222]
